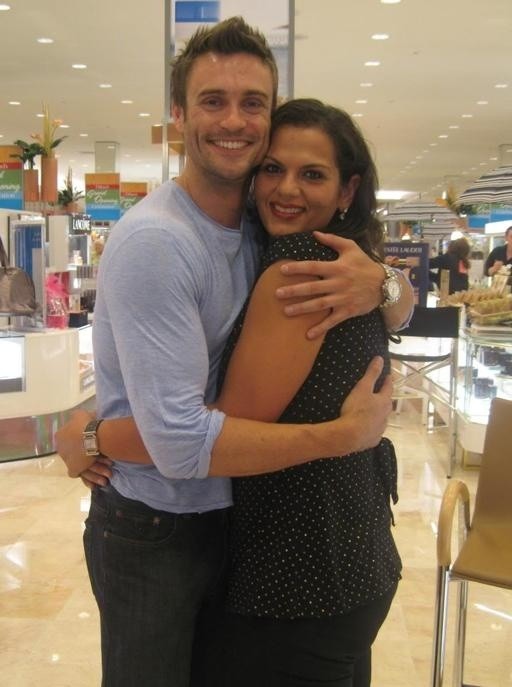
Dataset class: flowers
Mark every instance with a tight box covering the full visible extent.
[30,104,67,156]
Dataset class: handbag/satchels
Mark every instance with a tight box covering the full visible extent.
[0,240,36,317]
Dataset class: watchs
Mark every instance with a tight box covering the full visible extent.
[82,418,104,458]
[378,260,402,314]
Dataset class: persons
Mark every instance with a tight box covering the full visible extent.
[445,237,473,275]
[483,225,511,292]
[52,96,403,686]
[82,13,416,686]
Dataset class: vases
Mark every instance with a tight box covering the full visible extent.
[41,156,58,202]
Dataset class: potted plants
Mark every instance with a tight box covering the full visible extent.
[9,139,44,203]
[58,186,76,211]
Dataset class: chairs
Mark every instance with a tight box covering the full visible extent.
[433,396,512,687]
